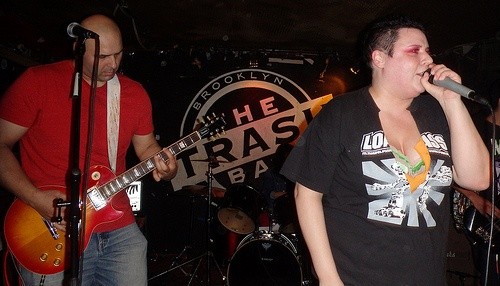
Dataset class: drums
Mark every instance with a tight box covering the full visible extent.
[227,231,303,286]
[217,182,265,235]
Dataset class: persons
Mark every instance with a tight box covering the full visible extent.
[451,83,500,286]
[279,20,491,286]
[190,144,315,261]
[0,13,177,286]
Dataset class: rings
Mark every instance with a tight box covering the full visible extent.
[484,213,489,217]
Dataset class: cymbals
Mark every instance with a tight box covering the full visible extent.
[182,183,226,198]
[191,159,228,163]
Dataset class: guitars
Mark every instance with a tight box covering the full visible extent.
[476,218,500,244]
[3,114,226,275]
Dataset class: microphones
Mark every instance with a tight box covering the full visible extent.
[422,69,488,106]
[66,22,99,39]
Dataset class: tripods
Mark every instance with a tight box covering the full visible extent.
[147,162,227,286]
